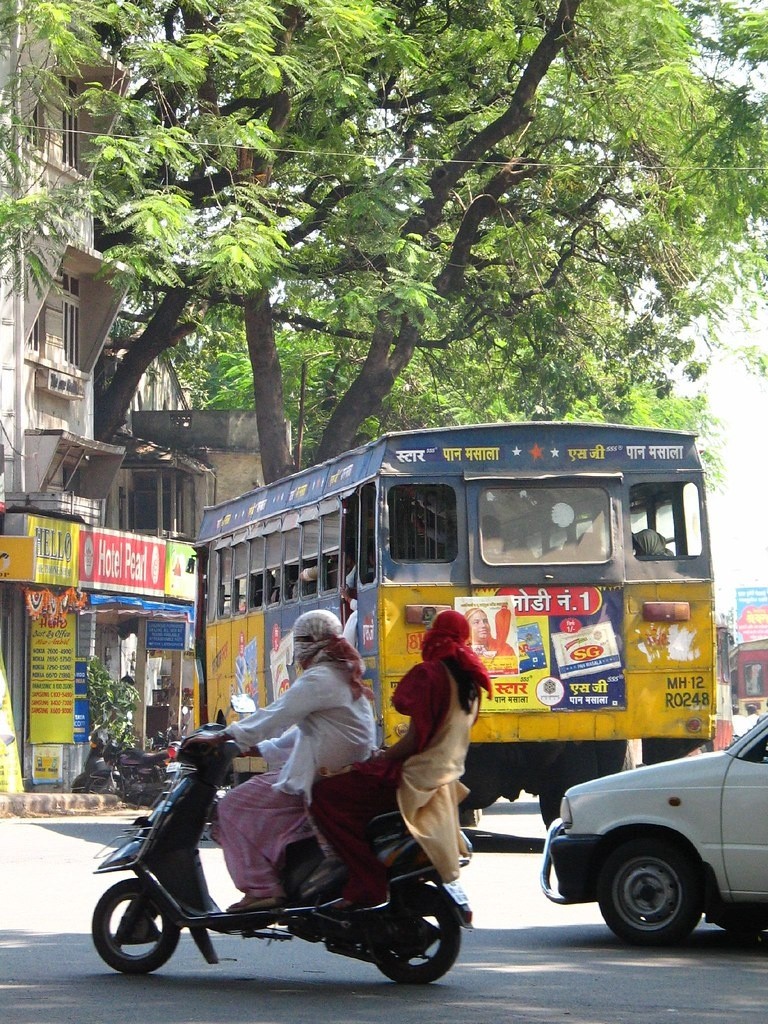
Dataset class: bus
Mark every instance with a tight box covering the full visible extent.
[186,420,717,836]
[643,625,768,765]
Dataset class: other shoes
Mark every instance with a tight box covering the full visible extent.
[306,858,350,883]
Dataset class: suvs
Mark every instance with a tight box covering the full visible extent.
[542,714,767,950]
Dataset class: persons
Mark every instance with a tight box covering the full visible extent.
[407,493,605,564]
[634,529,674,558]
[307,611,492,911]
[465,607,518,675]
[182,611,377,910]
[253,541,377,648]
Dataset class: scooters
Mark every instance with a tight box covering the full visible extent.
[90,694,477,986]
[71,706,190,808]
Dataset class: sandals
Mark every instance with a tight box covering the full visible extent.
[226,895,276,912]
